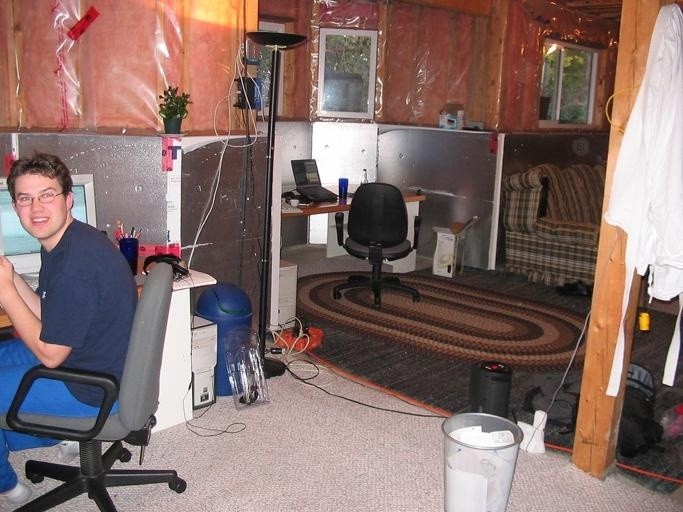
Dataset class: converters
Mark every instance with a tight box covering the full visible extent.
[234,76,262,110]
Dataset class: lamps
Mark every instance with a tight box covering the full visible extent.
[246,31,307,379]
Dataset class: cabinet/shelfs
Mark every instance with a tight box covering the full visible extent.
[148,266,217,436]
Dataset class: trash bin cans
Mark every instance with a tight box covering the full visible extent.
[441,413,523,512]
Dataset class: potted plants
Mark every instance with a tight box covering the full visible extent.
[159,85,194,134]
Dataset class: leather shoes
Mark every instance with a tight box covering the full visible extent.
[556,280,593,300]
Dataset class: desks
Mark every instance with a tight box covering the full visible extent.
[0,284,143,329]
[282,179,425,273]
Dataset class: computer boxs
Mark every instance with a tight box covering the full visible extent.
[278,258,298,331]
[190,312,218,410]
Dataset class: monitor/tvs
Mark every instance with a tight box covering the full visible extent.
[0,174,97,278]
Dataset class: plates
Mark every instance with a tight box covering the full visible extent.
[155,133,188,138]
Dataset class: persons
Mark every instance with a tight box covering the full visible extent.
[0,152,138,504]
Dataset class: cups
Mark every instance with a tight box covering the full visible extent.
[338,196,347,207]
[120,238,138,276]
[339,179,349,198]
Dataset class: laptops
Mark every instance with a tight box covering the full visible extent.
[291,159,338,201]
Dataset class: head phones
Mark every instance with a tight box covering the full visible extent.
[143,254,188,282]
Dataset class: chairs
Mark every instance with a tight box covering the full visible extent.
[0,261,187,512]
[333,182,422,311]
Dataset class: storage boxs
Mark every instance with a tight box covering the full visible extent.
[431,215,481,278]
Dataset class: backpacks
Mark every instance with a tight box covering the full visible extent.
[522,362,665,458]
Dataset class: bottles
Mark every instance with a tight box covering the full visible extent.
[360,169,369,186]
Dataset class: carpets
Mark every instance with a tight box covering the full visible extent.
[280,254,682,495]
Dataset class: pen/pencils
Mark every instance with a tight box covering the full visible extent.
[114,221,142,242]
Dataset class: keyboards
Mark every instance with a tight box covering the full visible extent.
[21,278,39,292]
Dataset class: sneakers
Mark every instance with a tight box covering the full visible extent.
[57,441,79,465]
[0,482,31,504]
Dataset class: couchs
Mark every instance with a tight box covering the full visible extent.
[503,162,608,292]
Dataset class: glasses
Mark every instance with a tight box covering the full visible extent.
[14,190,65,207]
